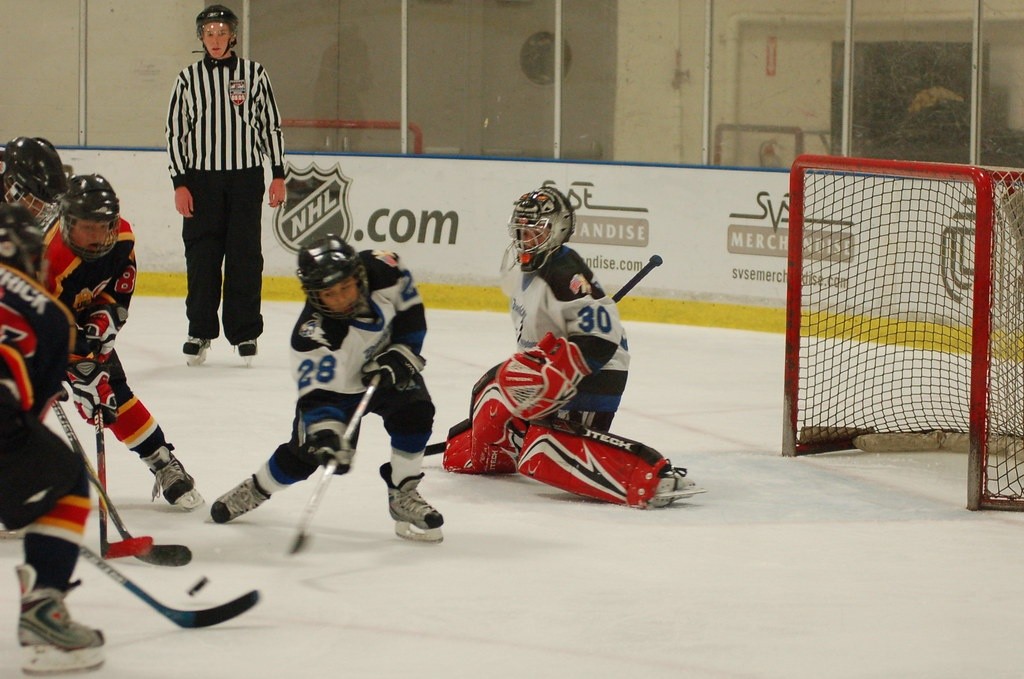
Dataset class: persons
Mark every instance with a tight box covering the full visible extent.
[209,240,445,541]
[0,135,204,675]
[164,4,287,366]
[442,186,706,508]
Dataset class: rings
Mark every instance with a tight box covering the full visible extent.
[278,202,280,204]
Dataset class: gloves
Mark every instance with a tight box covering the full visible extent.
[306,421,353,476]
[64,366,123,431]
[85,309,117,366]
[361,344,425,394]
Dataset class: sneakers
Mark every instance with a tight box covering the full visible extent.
[15,563,106,671]
[238,337,257,368]
[183,336,211,366]
[205,474,274,523]
[140,443,205,512]
[648,478,696,506]
[380,461,445,544]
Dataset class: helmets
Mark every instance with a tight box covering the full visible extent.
[500,186,578,273]
[0,136,70,230]
[195,3,239,49]
[58,173,121,260]
[0,203,45,280]
[295,232,369,322]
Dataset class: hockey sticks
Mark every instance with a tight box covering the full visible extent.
[423,253,664,458]
[80,544,262,629]
[288,372,383,556]
[50,400,194,567]
[92,407,155,559]
